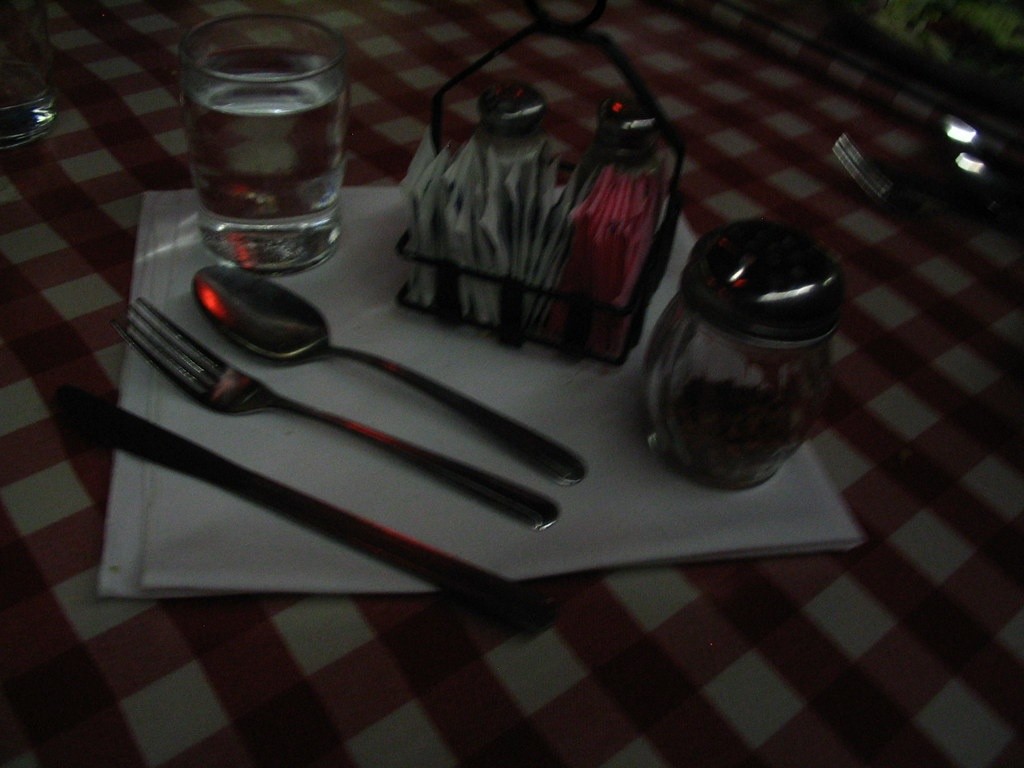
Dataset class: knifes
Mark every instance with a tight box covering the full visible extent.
[53,384,560,636]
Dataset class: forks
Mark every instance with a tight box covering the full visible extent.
[110,296,562,532]
[831,130,1024,232]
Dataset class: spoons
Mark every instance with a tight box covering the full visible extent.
[191,265,590,489]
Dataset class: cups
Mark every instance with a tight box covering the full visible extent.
[1,0,58,150]
[177,12,349,277]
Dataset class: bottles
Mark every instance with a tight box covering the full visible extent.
[641,218,847,490]
[571,94,675,204]
[466,81,552,183]
